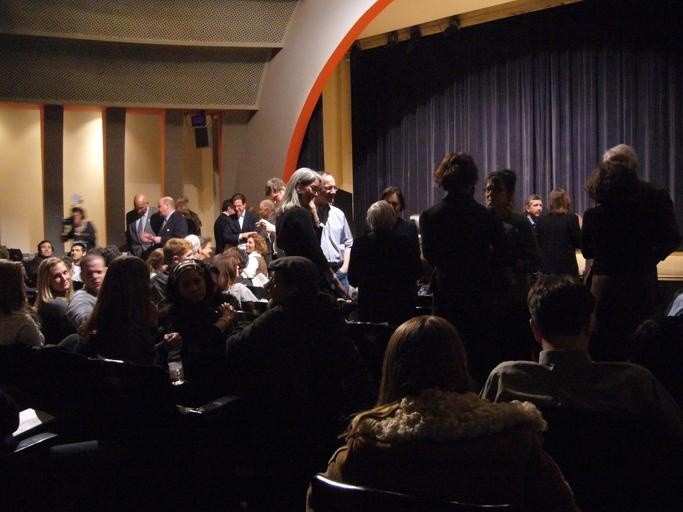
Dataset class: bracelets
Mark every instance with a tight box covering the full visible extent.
[218,317,230,329]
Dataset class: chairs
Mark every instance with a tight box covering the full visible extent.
[309,474,511,507]
[344,318,391,356]
[85,352,243,432]
[241,299,268,314]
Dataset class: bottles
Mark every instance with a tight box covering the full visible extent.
[167,337,185,386]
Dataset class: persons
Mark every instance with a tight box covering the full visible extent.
[379,186,418,228]
[222,193,260,244]
[175,195,202,237]
[71,243,86,265]
[602,144,674,210]
[25,240,54,287]
[0,258,79,346]
[150,238,196,304]
[347,200,419,324]
[74,255,186,414]
[184,234,207,261]
[484,169,543,318]
[227,256,376,409]
[240,233,269,277]
[34,257,75,332]
[255,178,288,257]
[276,167,353,302]
[259,200,276,253]
[101,244,135,266]
[199,238,213,258]
[580,161,674,311]
[306,315,578,511]
[63,207,95,251]
[535,187,582,275]
[214,199,235,254]
[477,275,683,438]
[522,194,544,227]
[139,196,188,247]
[309,171,354,290]
[126,194,160,256]
[223,247,253,286]
[419,152,507,317]
[165,259,236,388]
[146,248,168,280]
[212,253,269,308]
[65,253,106,329]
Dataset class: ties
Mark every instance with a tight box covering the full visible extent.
[138,216,143,239]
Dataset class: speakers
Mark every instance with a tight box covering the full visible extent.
[195,127,209,148]
